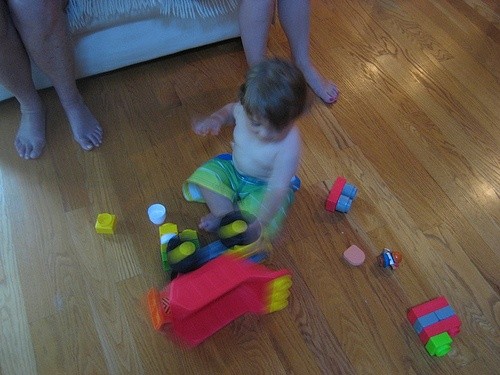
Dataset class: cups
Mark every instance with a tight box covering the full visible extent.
[147,203,167,225]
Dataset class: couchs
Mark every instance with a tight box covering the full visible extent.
[0,0,278,102]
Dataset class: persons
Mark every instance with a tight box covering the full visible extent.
[0,0,104,160]
[181,56,310,261]
[239,0,342,103]
